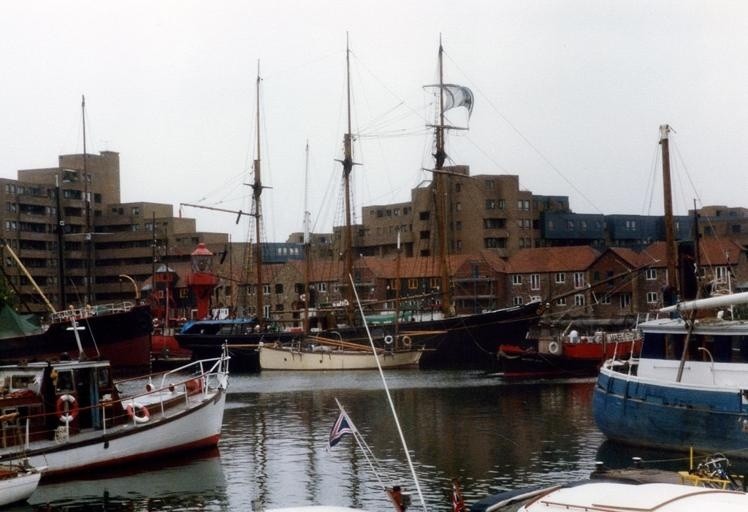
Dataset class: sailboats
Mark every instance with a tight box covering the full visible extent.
[0,30,748,372]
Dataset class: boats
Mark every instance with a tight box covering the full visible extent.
[590,284,748,457]
[0,456,49,508]
[471,470,748,512]
[0,317,232,476]
